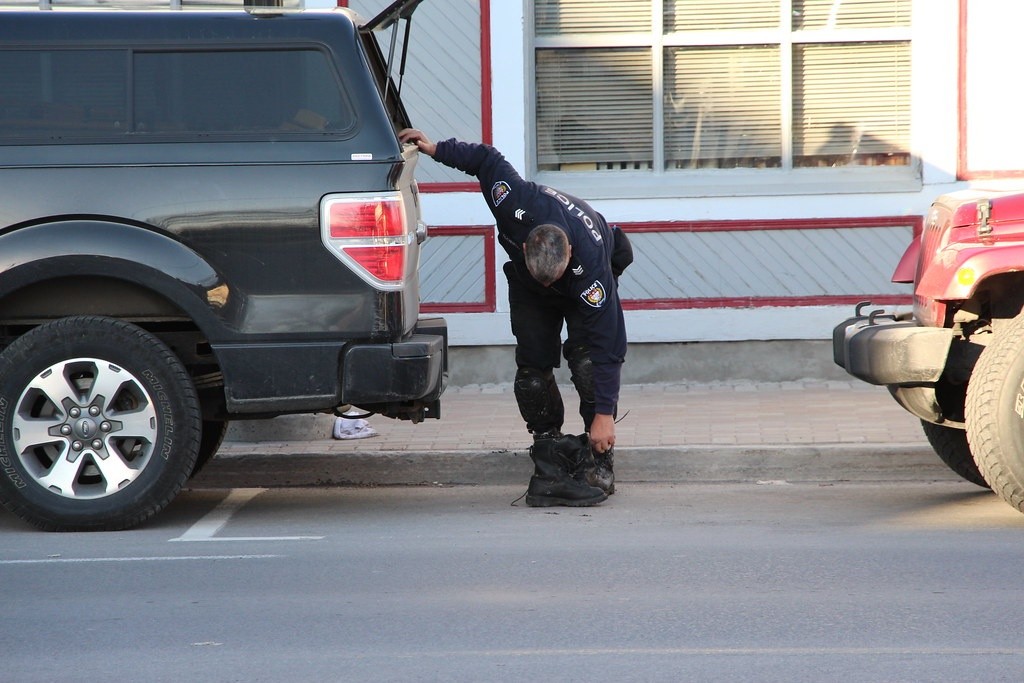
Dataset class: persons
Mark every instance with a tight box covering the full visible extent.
[396,118,628,495]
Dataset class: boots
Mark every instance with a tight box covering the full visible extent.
[526,430,617,507]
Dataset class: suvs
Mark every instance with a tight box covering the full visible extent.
[2,2,451,534]
[832,175,1024,521]
